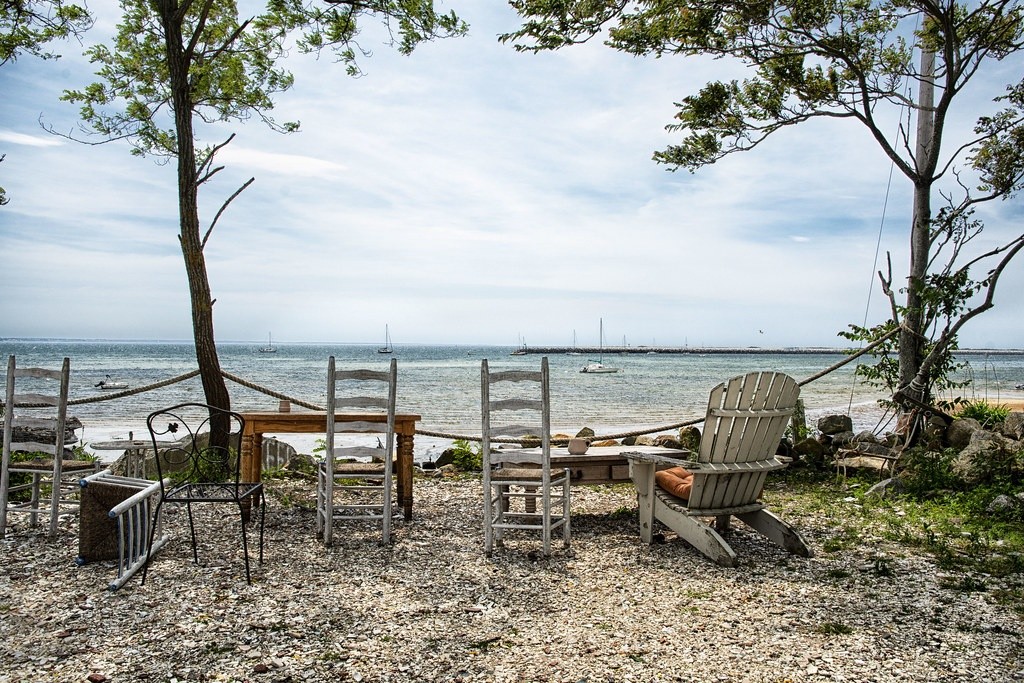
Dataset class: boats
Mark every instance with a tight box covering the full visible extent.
[95,375,129,389]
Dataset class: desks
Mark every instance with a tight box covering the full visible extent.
[494,445,692,512]
[235,409,421,520]
[89,440,183,478]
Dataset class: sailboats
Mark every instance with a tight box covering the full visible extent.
[566,328,584,356]
[580,317,619,372]
[378,323,393,353]
[260,331,277,352]
[510,332,527,355]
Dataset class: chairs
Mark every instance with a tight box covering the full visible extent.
[316,356,398,546]
[1,354,100,541]
[140,402,266,587]
[76,469,170,590]
[620,370,814,565]
[481,357,571,556]
